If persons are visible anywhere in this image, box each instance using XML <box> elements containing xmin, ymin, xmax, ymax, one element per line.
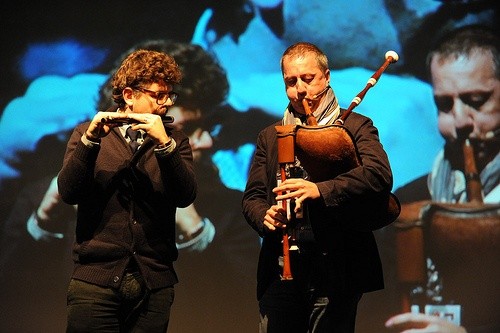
<box><xmin>56</xmin><ymin>50</ymin><xmax>196</xmax><ymax>332</ymax></box>
<box><xmin>243</xmin><ymin>42</ymin><xmax>393</xmax><ymax>332</ymax></box>
<box><xmin>0</xmin><ymin>38</ymin><xmax>283</xmax><ymax>333</ymax></box>
<box><xmin>352</xmin><ymin>0</ymin><xmax>500</xmax><ymax>333</ymax></box>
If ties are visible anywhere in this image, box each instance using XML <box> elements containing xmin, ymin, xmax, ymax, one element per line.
<box><xmin>127</xmin><ymin>126</ymin><xmax>140</xmax><ymax>155</ymax></box>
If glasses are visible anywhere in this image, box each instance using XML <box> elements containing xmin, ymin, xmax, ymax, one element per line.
<box><xmin>136</xmin><ymin>85</ymin><xmax>178</xmax><ymax>104</ymax></box>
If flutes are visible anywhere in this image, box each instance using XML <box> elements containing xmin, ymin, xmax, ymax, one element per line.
<box><xmin>396</xmin><ymin>138</ymin><xmax>500</xmax><ymax>333</ymax></box>
<box><xmin>275</xmin><ymin>50</ymin><xmax>399</xmax><ymax>282</ymax></box>
<box><xmin>101</xmin><ymin>116</ymin><xmax>175</xmax><ymax>124</ymax></box>
